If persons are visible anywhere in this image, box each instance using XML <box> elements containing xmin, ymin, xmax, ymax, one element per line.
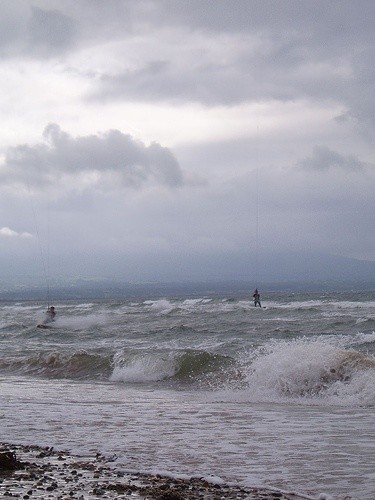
<box><xmin>253</xmin><ymin>289</ymin><xmax>262</xmax><ymax>307</ymax></box>
<box><xmin>40</xmin><ymin>307</ymin><xmax>56</xmax><ymax>326</ymax></box>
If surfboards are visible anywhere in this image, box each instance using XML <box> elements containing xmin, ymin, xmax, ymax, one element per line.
<box><xmin>36</xmin><ymin>324</ymin><xmax>45</xmax><ymax>328</ymax></box>
